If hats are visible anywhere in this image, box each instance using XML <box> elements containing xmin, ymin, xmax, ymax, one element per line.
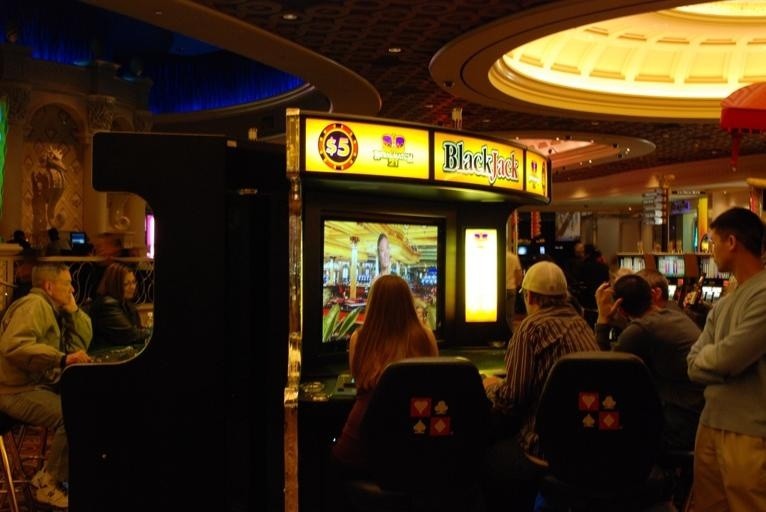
<box><xmin>519</xmin><ymin>261</ymin><xmax>568</xmax><ymax>295</ymax></box>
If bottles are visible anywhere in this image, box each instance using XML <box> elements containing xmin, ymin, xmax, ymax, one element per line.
<box><xmin>686</xmin><ymin>272</ymin><xmax>707</xmax><ymax>310</ymax></box>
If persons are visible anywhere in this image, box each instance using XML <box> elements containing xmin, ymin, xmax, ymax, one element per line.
<box><xmin>327</xmin><ymin>272</ymin><xmax>441</xmax><ymax>456</ymax></box>
<box><xmin>87</xmin><ymin>262</ymin><xmax>151</xmax><ymax>351</ymax></box>
<box><xmin>43</xmin><ymin>226</ymin><xmax>66</xmax><ymax>255</ymax></box>
<box><xmin>5</xmin><ymin>229</ymin><xmax>31</xmax><ymax>249</ymax></box>
<box><xmin>683</xmin><ymin>206</ymin><xmax>765</xmax><ymax>512</ymax></box>
<box><xmin>480</xmin><ymin>261</ymin><xmax>601</xmax><ymax>461</ymax></box>
<box><xmin>593</xmin><ymin>268</ymin><xmax>702</xmax><ymax>417</ymax></box>
<box><xmin>93</xmin><ymin>230</ymin><xmax>138</xmax><ymax>271</ymax></box>
<box><xmin>0</xmin><ymin>261</ymin><xmax>95</xmax><ymax>508</ymax></box>
<box><xmin>375</xmin><ymin>230</ymin><xmax>393</xmax><ymax>275</ymax></box>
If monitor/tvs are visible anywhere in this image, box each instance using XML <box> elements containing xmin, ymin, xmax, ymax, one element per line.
<box><xmin>323</xmin><ymin>214</ymin><xmax>445</xmax><ymax>349</ymax></box>
<box><xmin>70</xmin><ymin>233</ymin><xmax>85</xmax><ymax>248</ymax></box>
<box><xmin>462</xmin><ymin>225</ymin><xmax>499</xmax><ymax>323</ymax></box>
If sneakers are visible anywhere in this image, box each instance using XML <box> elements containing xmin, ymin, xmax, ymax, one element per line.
<box><xmin>31</xmin><ymin>469</ymin><xmax>69</xmax><ymax>508</ymax></box>
